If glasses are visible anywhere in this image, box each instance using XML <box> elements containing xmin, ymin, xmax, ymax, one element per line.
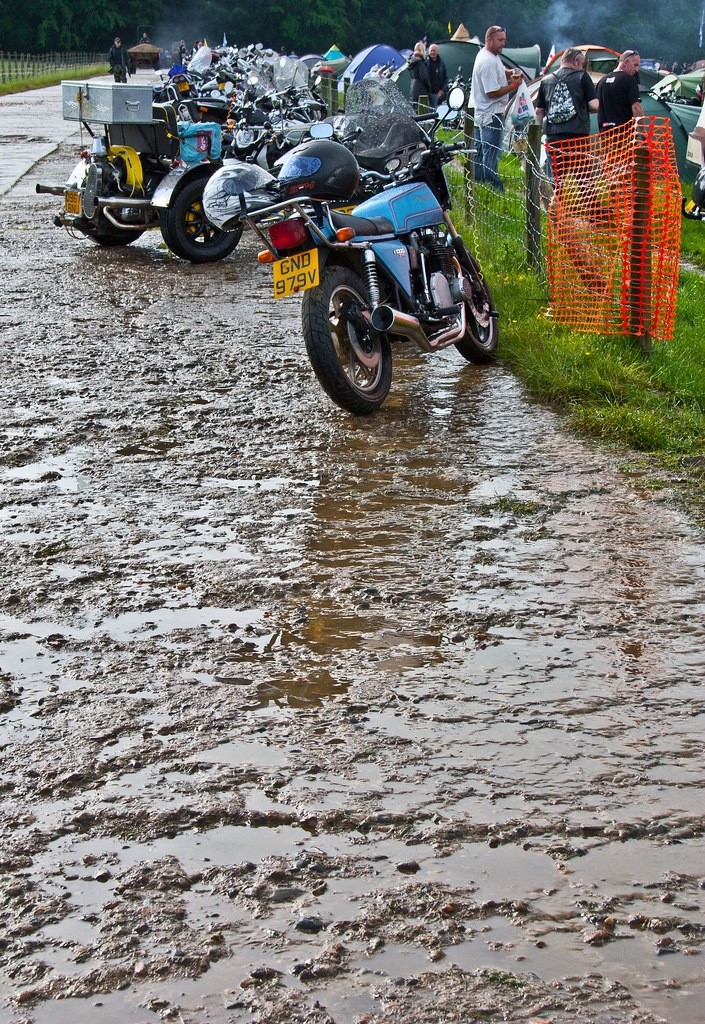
<box><xmin>622</xmin><ymin>49</ymin><xmax>639</xmax><ymax>62</ymax></box>
<box><xmin>561</xmin><ymin>49</ymin><xmax>582</xmax><ymax>64</ymax></box>
<box><xmin>486</xmin><ymin>27</ymin><xmax>506</xmax><ymax>38</ymax></box>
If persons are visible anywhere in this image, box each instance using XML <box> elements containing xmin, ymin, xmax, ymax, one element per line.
<box><xmin>178</xmin><ymin>40</ymin><xmax>186</xmax><ymax>66</ymax></box>
<box><xmin>108</xmin><ymin>37</ymin><xmax>132</xmax><ymax>83</ymax></box>
<box><xmin>289</xmin><ymin>54</ymin><xmax>298</xmax><ymax>59</ymax></box>
<box><xmin>594</xmin><ymin>50</ymin><xmax>646</xmax><ymax>209</ymax></box>
<box><xmin>425</xmin><ymin>44</ymin><xmax>449</xmax><ymax>106</ymax></box>
<box><xmin>535</xmin><ymin>45</ymin><xmax>616</xmax><ymax>226</ymax></box>
<box><xmin>139</xmin><ymin>32</ymin><xmax>150</xmax><ymax>45</ymax></box>
<box><xmin>348</xmin><ymin>54</ymin><xmax>353</xmax><ymax>61</ymax></box>
<box><xmin>468</xmin><ymin>24</ymin><xmax>524</xmax><ymax>193</ymax></box>
<box><xmin>409</xmin><ymin>42</ymin><xmax>436</xmax><ymax>112</ymax></box>
<box><xmin>192</xmin><ymin>39</ymin><xmax>199</xmax><ymax>54</ymax></box>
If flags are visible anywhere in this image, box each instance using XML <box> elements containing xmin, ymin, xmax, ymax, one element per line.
<box><xmin>223</xmin><ymin>34</ymin><xmax>228</xmax><ymax>47</ymax></box>
<box><xmin>203</xmin><ymin>39</ymin><xmax>207</xmax><ymax>46</ymax></box>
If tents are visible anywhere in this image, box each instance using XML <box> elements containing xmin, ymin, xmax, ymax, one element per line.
<box><xmin>649</xmin><ymin>69</ymin><xmax>705</xmax><ymax>108</ymax></box>
<box><xmin>503</xmin><ymin>67</ymin><xmax>701</xmax><ymax>192</ymax></box>
<box><xmin>451</xmin><ymin>23</ymin><xmax>482</xmax><ymax>46</ymax></box>
<box><xmin>311</xmin><ymin>56</ymin><xmax>352</xmax><ymax>82</ymax></box>
<box><xmin>337</xmin><ymin>43</ymin><xmax>408</xmax><ymax>110</ymax></box>
<box><xmin>299</xmin><ymin>52</ymin><xmax>327</xmax><ymax>67</ymax></box>
<box><xmin>390</xmin><ymin>38</ymin><xmax>542</xmax><ymax>122</ymax></box>
<box><xmin>543</xmin><ymin>42</ymin><xmax>625</xmax><ymax>76</ymax></box>
<box><xmin>399</xmin><ymin>47</ymin><xmax>414</xmax><ymax>57</ymax></box>
<box><xmin>323</xmin><ymin>43</ymin><xmax>348</xmax><ymax>61</ymax></box>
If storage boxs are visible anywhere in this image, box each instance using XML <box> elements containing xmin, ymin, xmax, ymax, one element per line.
<box><xmin>60</xmin><ymin>79</ymin><xmax>155</xmax><ymax>124</ymax></box>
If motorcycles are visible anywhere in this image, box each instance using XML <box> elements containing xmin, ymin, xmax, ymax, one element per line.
<box><xmin>241</xmin><ymin>82</ymin><xmax>506</xmax><ymax>414</ymax></box>
<box><xmin>35</xmin><ymin>33</ymin><xmax>462</xmax><ymax>263</ymax></box>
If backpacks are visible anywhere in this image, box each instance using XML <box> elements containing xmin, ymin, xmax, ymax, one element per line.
<box><xmin>546</xmin><ymin>67</ymin><xmax>583</xmax><ymax>124</ymax></box>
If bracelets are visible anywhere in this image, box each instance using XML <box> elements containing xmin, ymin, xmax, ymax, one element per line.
<box><xmin>509</xmin><ymin>82</ymin><xmax>515</xmax><ymax>90</ymax></box>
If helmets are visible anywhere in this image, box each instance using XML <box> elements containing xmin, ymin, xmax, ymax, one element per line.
<box><xmin>202</xmin><ymin>160</ymin><xmax>279</xmax><ymax>234</ymax></box>
<box><xmin>276</xmin><ymin>138</ymin><xmax>361</xmax><ymax>206</ymax></box>
